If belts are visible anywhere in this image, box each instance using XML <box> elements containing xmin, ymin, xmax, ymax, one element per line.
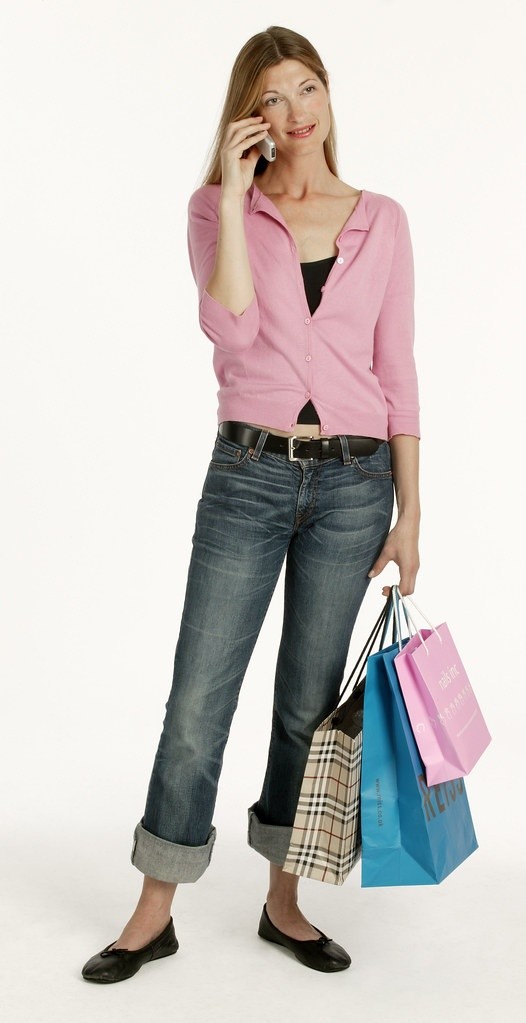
<box><xmin>218</xmin><ymin>422</ymin><xmax>381</xmax><ymax>460</ymax></box>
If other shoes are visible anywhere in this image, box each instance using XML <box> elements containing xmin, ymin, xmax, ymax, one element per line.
<box><xmin>81</xmin><ymin>914</ymin><xmax>180</xmax><ymax>983</ymax></box>
<box><xmin>257</xmin><ymin>903</ymin><xmax>352</xmax><ymax>973</ymax></box>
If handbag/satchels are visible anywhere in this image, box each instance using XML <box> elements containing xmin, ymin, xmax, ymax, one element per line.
<box><xmin>392</xmin><ymin>584</ymin><xmax>492</xmax><ymax>787</ymax></box>
<box><xmin>360</xmin><ymin>589</ymin><xmax>481</xmax><ymax>889</ymax></box>
<box><xmin>282</xmin><ymin>589</ymin><xmax>399</xmax><ymax>888</ymax></box>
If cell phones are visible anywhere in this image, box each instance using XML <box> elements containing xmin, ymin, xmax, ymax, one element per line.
<box><xmin>250</xmin><ymin>130</ymin><xmax>276</xmax><ymax>161</ymax></box>
